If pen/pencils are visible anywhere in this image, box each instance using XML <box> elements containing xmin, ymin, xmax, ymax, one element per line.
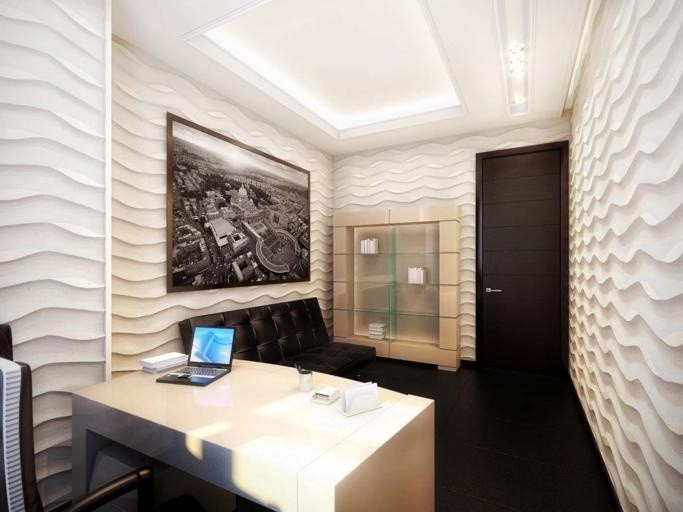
<box><xmin>294</xmin><ymin>363</ymin><xmax>302</xmax><ymax>373</ymax></box>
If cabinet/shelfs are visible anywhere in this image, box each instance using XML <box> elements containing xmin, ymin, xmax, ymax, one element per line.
<box><xmin>333</xmin><ymin>205</ymin><xmax>461</xmax><ymax>372</ymax></box>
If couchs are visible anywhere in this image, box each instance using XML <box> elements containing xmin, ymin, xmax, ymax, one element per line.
<box><xmin>178</xmin><ymin>296</ymin><xmax>376</xmax><ymax>377</ymax></box>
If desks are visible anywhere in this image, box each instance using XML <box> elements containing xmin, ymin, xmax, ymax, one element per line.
<box><xmin>71</xmin><ymin>358</ymin><xmax>437</xmax><ymax>512</ymax></box>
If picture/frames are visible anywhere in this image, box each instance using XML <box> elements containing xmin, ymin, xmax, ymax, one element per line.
<box><xmin>167</xmin><ymin>112</ymin><xmax>310</xmax><ymax>293</ymax></box>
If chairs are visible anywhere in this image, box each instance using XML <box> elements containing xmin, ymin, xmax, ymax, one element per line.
<box><xmin>1</xmin><ymin>323</ymin><xmax>205</xmax><ymax>512</ymax></box>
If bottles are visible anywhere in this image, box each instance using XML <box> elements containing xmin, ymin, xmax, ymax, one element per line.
<box><xmin>296</xmin><ymin>363</ymin><xmax>313</xmax><ymax>391</ymax></box>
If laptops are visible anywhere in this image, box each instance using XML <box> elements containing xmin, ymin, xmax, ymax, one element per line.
<box><xmin>155</xmin><ymin>326</ymin><xmax>237</xmax><ymax>386</ymax></box>
